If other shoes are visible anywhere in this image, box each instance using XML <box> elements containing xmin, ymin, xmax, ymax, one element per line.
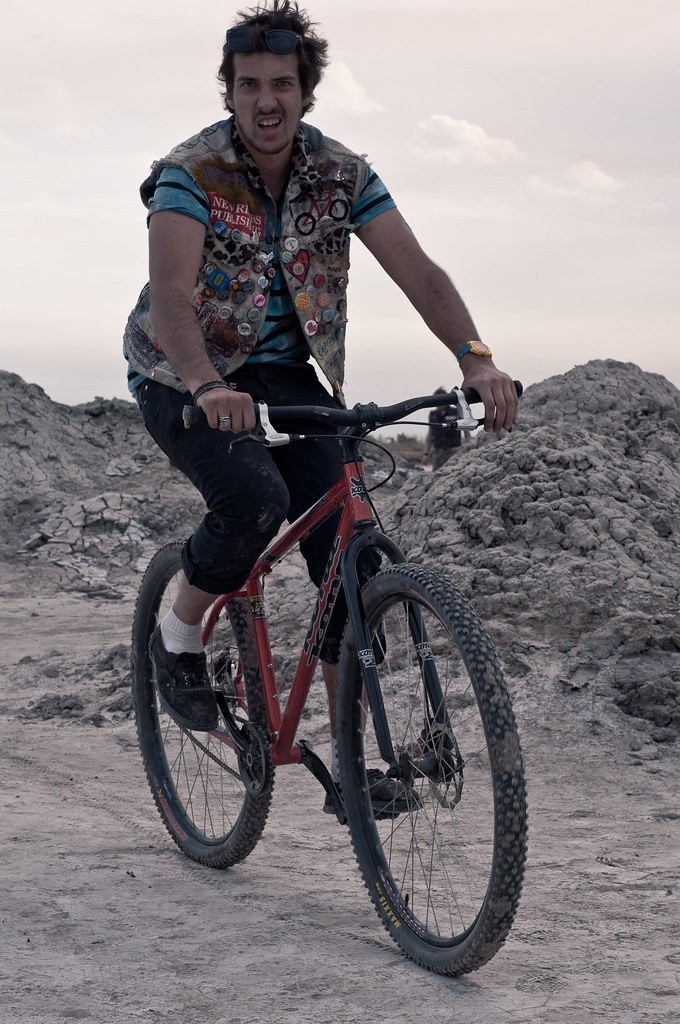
<box><xmin>148</xmin><ymin>623</ymin><xmax>219</xmax><ymax>731</ymax></box>
<box><xmin>322</xmin><ymin>769</ymin><xmax>424</xmax><ymax>813</ymax></box>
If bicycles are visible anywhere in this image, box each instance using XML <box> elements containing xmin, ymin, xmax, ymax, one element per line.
<box><xmin>130</xmin><ymin>380</ymin><xmax>527</xmax><ymax>977</ymax></box>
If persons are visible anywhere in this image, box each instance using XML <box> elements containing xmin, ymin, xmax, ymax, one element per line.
<box><xmin>421</xmin><ymin>387</ymin><xmax>463</xmax><ymax>471</ymax></box>
<box><xmin>122</xmin><ymin>0</ymin><xmax>518</xmax><ymax>813</ymax></box>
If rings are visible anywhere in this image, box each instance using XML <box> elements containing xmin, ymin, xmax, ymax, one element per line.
<box><xmin>219</xmin><ymin>417</ymin><xmax>232</xmax><ymax>424</ymax></box>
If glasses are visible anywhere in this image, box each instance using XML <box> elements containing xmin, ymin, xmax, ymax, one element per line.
<box><xmin>226</xmin><ymin>28</ymin><xmax>302</xmax><ymax>57</ymax></box>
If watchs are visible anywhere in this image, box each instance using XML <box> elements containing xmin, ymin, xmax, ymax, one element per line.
<box><xmin>456</xmin><ymin>341</ymin><xmax>492</xmax><ymax>359</ymax></box>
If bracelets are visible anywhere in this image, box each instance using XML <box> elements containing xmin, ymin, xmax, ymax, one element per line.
<box><xmin>194</xmin><ymin>380</ymin><xmax>233</xmax><ymax>403</ymax></box>
<box><xmin>424</xmin><ymin>453</ymin><xmax>428</xmax><ymax>456</ymax></box>
